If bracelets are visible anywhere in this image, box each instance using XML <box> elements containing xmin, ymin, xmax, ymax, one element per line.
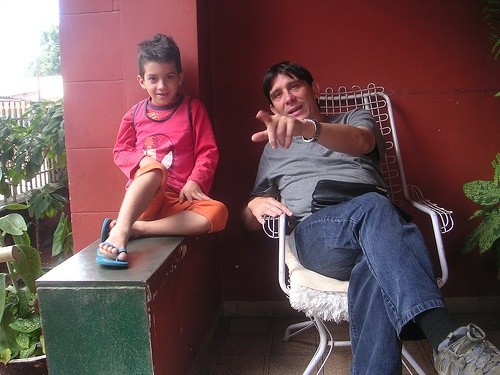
<box><xmin>301</xmin><ymin>119</ymin><xmax>321</xmax><ymax>143</ymax></box>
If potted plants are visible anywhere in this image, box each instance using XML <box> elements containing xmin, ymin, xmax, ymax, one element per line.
<box><xmin>0</xmin><ymin>204</ymin><xmax>49</xmax><ymax>375</ymax></box>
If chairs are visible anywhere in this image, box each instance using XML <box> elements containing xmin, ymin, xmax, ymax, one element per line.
<box><xmin>262</xmin><ymin>82</ymin><xmax>454</xmax><ymax>375</ymax></box>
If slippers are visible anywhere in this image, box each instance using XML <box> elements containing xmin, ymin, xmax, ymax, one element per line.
<box><xmin>101</xmin><ymin>217</ymin><xmax>115</xmax><ymax>242</ymax></box>
<box><xmin>96</xmin><ymin>241</ymin><xmax>129</xmax><ymax>267</ymax></box>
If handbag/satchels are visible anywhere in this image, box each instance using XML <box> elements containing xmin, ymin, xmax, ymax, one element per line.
<box><xmin>311</xmin><ymin>180</ymin><xmax>389</xmax><ymax>213</ymax></box>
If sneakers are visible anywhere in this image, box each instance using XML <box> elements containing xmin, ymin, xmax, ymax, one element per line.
<box><xmin>430</xmin><ymin>323</ymin><xmax>500</xmax><ymax>374</ymax></box>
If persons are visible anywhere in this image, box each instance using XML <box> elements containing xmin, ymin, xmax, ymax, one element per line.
<box><xmin>95</xmin><ymin>33</ymin><xmax>228</xmax><ymax>267</ymax></box>
<box><xmin>241</xmin><ymin>59</ymin><xmax>500</xmax><ymax>375</ymax></box>
